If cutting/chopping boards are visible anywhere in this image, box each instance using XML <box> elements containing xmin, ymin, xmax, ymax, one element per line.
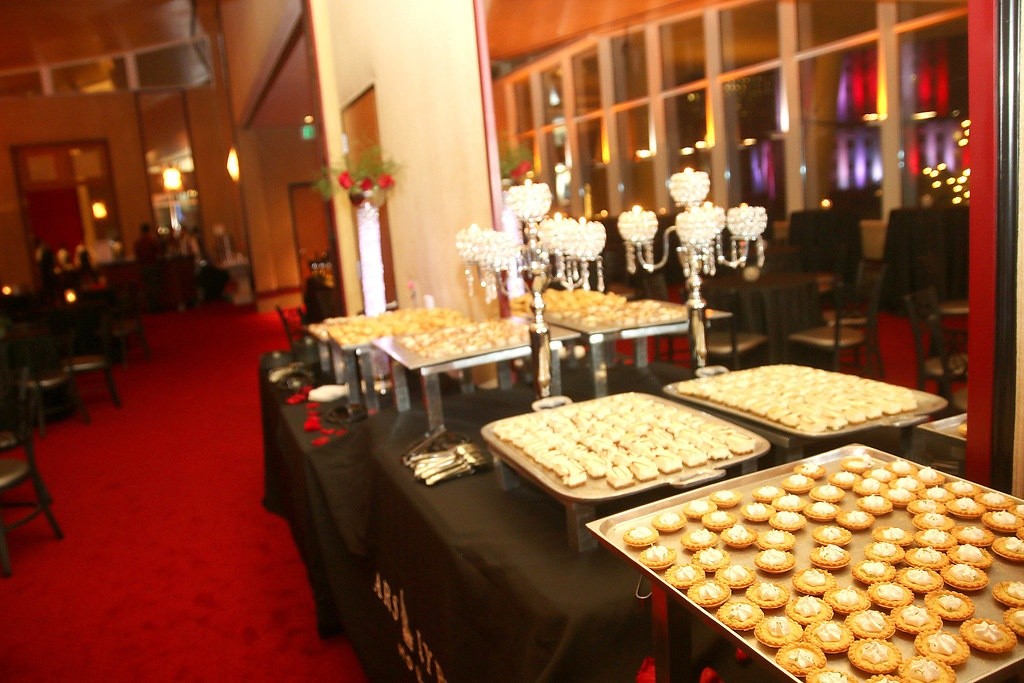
<box><xmin>481</xmin><ymin>391</ymin><xmax>771</xmax><ymax>501</ymax></box>
<box><xmin>664</xmin><ymin>364</ymin><xmax>948</xmax><ymax>437</ymax></box>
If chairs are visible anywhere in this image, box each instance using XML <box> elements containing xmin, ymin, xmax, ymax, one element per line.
<box><xmin>600</xmin><ymin>200</ymin><xmax>980</xmax><ymax>416</ymax></box>
<box><xmin>0</xmin><ymin>282</ymin><xmax>147</xmax><ymax>577</ymax></box>
<box><xmin>274</xmin><ymin>303</ymin><xmax>305</xmax><ymax>345</ymax></box>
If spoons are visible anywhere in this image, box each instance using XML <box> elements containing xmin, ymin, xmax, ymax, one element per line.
<box><xmin>407</xmin><ymin>444</ymin><xmax>487</xmax><ymax>485</ymax></box>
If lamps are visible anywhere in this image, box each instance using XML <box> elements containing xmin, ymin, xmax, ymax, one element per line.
<box><xmin>623</xmin><ymin>163</ymin><xmax>768</xmax><ymax>375</ymax></box>
<box><xmin>456</xmin><ymin>165</ymin><xmax>607</xmax><ymax>410</ymax></box>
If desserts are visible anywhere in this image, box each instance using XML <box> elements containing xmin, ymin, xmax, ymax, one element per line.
<box><xmin>676</xmin><ymin>365</ymin><xmax>920</xmax><ymax>432</ymax></box>
<box><xmin>491</xmin><ymin>391</ymin><xmax>754</xmax><ymax>491</ymax></box>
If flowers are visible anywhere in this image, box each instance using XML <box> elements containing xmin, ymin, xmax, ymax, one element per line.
<box><xmin>316</xmin><ymin>147</ymin><xmax>404</xmax><ymax>209</ymax></box>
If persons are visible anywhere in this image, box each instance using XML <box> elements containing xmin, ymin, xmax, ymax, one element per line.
<box><xmin>28</xmin><ymin>222</ymin><xmax>157</xmax><ymax>317</ymax></box>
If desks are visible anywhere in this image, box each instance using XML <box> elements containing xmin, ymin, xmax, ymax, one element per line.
<box><xmin>260</xmin><ymin>327</ymin><xmax>828</xmax><ymax>683</ymax></box>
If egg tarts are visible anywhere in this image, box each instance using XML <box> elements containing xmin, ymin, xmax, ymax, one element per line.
<box><xmin>624</xmin><ymin>454</ymin><xmax>1024</xmax><ymax>683</ymax></box>
